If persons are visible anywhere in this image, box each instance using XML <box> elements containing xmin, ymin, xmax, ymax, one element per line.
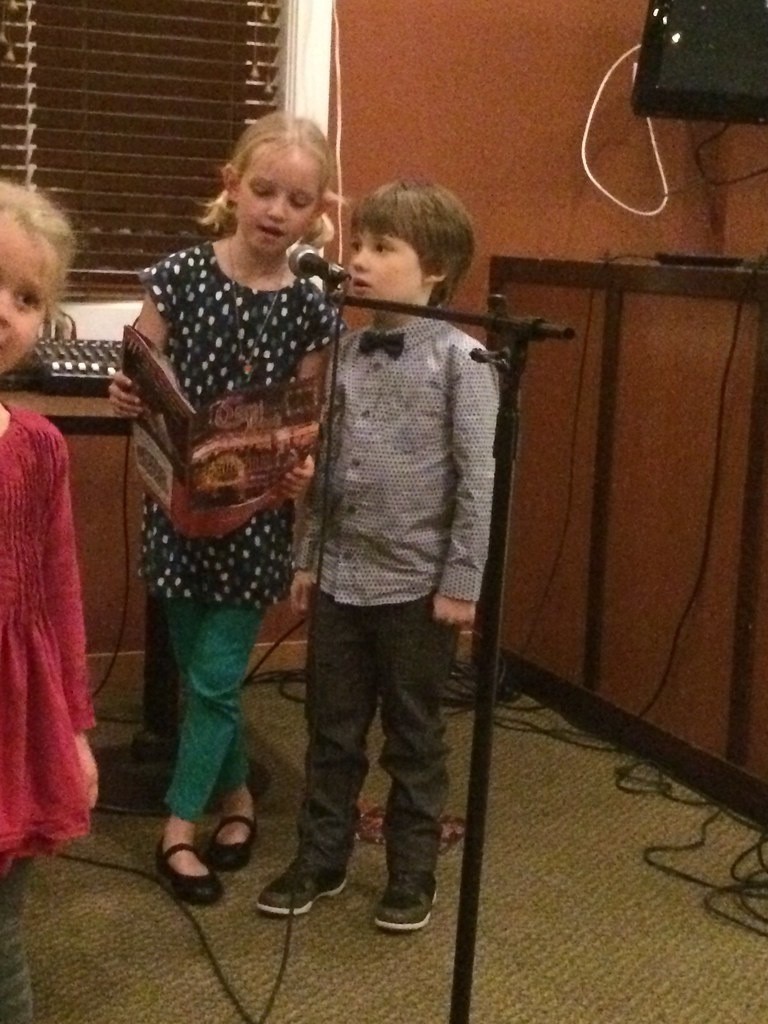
<box><xmin>0</xmin><ymin>177</ymin><xmax>98</xmax><ymax>1024</ymax></box>
<box><xmin>106</xmin><ymin>111</ymin><xmax>347</xmax><ymax>905</ymax></box>
<box><xmin>252</xmin><ymin>179</ymin><xmax>498</xmax><ymax>936</ymax></box>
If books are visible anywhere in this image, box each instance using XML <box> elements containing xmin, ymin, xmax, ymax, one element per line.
<box><xmin>123</xmin><ymin>323</ymin><xmax>329</xmax><ymax>539</ymax></box>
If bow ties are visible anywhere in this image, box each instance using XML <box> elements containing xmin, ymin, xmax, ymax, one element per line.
<box><xmin>354</xmin><ymin>329</ymin><xmax>403</xmax><ymax>358</ymax></box>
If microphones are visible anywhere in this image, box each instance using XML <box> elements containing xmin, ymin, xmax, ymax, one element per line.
<box><xmin>288</xmin><ymin>244</ymin><xmax>351</xmax><ymax>283</ymax></box>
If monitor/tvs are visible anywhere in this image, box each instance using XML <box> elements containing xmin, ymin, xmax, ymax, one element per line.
<box><xmin>630</xmin><ymin>2</ymin><xmax>767</xmax><ymax>125</ymax></box>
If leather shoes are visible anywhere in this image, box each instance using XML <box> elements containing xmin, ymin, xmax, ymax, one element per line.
<box><xmin>157</xmin><ymin>836</ymin><xmax>224</xmax><ymax>904</ymax></box>
<box><xmin>210</xmin><ymin>810</ymin><xmax>255</xmax><ymax>870</ymax></box>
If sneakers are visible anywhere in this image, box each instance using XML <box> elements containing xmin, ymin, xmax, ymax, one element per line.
<box><xmin>259</xmin><ymin>859</ymin><xmax>349</xmax><ymax>917</ymax></box>
<box><xmin>374</xmin><ymin>876</ymin><xmax>438</xmax><ymax>929</ymax></box>
